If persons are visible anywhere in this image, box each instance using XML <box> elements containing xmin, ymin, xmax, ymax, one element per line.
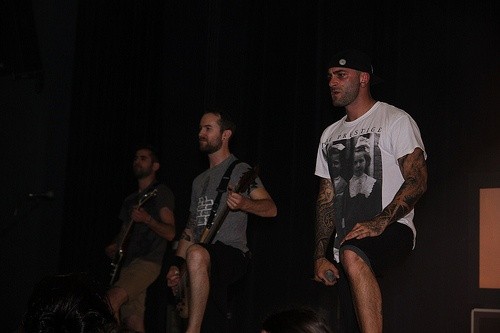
<box><xmin>24</xmin><ymin>272</ymin><xmax>120</xmax><ymax>333</ymax></box>
<box><xmin>166</xmin><ymin>106</ymin><xmax>278</xmax><ymax>333</ymax></box>
<box><xmin>101</xmin><ymin>146</ymin><xmax>176</xmax><ymax>333</ymax></box>
<box><xmin>258</xmin><ymin>305</ymin><xmax>331</xmax><ymax>333</ymax></box>
<box><xmin>311</xmin><ymin>52</ymin><xmax>428</xmax><ymax>333</ymax></box>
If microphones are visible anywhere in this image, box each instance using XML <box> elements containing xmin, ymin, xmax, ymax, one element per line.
<box><xmin>325</xmin><ymin>271</ymin><xmax>335</xmax><ymax>282</ymax></box>
<box><xmin>29</xmin><ymin>191</ymin><xmax>54</xmax><ymax>199</ymax></box>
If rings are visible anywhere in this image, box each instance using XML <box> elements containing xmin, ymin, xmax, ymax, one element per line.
<box><xmin>134</xmin><ymin>215</ymin><xmax>137</xmax><ymax>218</ymax></box>
<box><xmin>365</xmin><ymin>233</ymin><xmax>370</xmax><ymax>237</ymax></box>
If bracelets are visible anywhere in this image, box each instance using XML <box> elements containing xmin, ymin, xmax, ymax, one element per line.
<box><xmin>313</xmin><ymin>255</ymin><xmax>324</xmax><ymax>260</ymax></box>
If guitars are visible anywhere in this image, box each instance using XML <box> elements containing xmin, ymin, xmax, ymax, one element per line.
<box><xmin>172</xmin><ymin>164</ymin><xmax>262</xmax><ymax>318</ymax></box>
<box><xmin>104</xmin><ymin>188</ymin><xmax>158</xmax><ymax>284</ymax></box>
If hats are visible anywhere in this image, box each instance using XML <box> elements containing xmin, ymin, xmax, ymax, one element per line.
<box><xmin>329</xmin><ymin>53</ymin><xmax>369</xmax><ymax>74</ymax></box>
